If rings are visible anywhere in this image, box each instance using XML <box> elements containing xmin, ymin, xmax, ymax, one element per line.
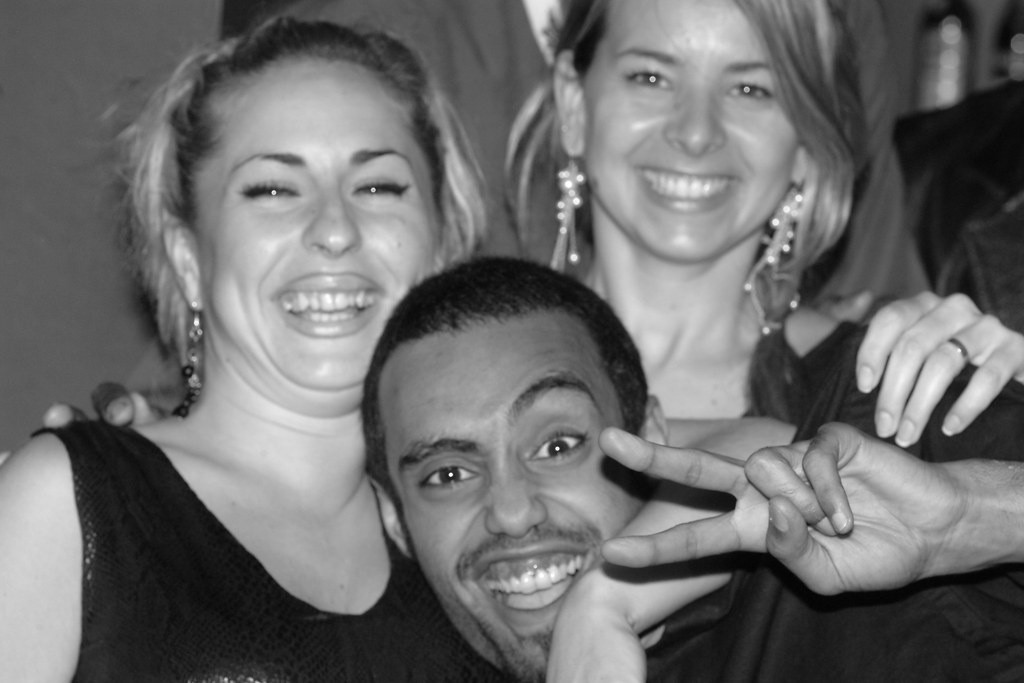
<box><xmin>947</xmin><ymin>338</ymin><xmax>969</xmax><ymax>363</ymax></box>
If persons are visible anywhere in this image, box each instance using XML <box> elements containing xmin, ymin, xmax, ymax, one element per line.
<box><xmin>41</xmin><ymin>0</ymin><xmax>1024</xmax><ymax>683</ymax></box>
<box><xmin>0</xmin><ymin>15</ymin><xmax>808</xmax><ymax>683</ymax></box>
